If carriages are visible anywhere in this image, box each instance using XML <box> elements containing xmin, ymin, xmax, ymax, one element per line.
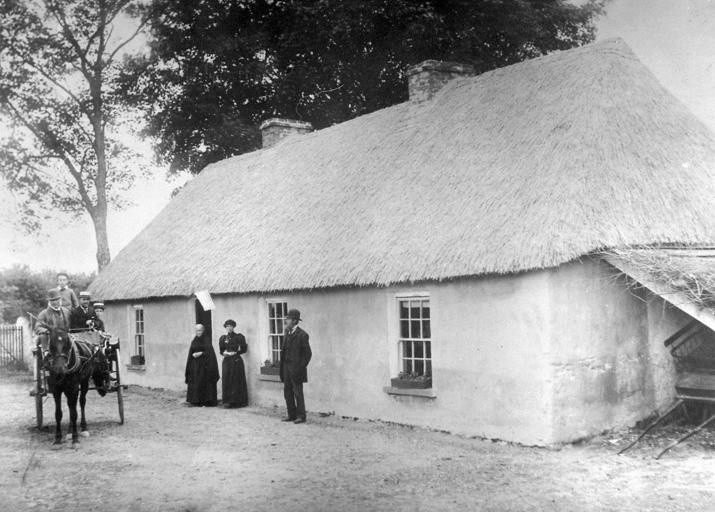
<box><xmin>31</xmin><ymin>328</ymin><xmax>125</xmax><ymax>447</ymax></box>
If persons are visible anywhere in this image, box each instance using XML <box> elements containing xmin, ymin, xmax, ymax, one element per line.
<box><xmin>72</xmin><ymin>291</ymin><xmax>104</xmax><ymax>332</ymax></box>
<box><xmin>184</xmin><ymin>323</ymin><xmax>220</xmax><ymax>407</ymax></box>
<box><xmin>34</xmin><ymin>287</ymin><xmax>72</xmax><ymax>336</ymax></box>
<box><xmin>56</xmin><ymin>272</ymin><xmax>79</xmax><ymax>310</ymax></box>
<box><xmin>278</xmin><ymin>308</ymin><xmax>314</xmax><ymax>425</ymax></box>
<box><xmin>92</xmin><ymin>302</ymin><xmax>106</xmax><ymax>320</ymax></box>
<box><xmin>218</xmin><ymin>318</ymin><xmax>248</xmax><ymax>409</ymax></box>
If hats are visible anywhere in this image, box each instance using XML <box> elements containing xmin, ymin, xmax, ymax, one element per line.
<box><xmin>44</xmin><ymin>288</ymin><xmax>61</xmax><ymax>301</ymax></box>
<box><xmin>285</xmin><ymin>309</ymin><xmax>302</xmax><ymax>321</ymax></box>
<box><xmin>223</xmin><ymin>319</ymin><xmax>236</xmax><ymax>328</ymax></box>
<box><xmin>92</xmin><ymin>302</ymin><xmax>106</xmax><ymax>310</ymax></box>
<box><xmin>77</xmin><ymin>291</ymin><xmax>92</xmax><ymax>300</ymax></box>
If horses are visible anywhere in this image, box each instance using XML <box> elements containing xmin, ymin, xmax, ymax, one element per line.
<box><xmin>44</xmin><ymin>324</ymin><xmax>94</xmax><ymax>450</ymax></box>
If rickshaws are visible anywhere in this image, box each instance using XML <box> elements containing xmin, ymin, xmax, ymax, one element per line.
<box><xmin>617</xmin><ymin>318</ymin><xmax>715</xmax><ymax>465</ymax></box>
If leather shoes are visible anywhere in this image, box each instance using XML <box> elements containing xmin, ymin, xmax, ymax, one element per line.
<box><xmin>281</xmin><ymin>416</ymin><xmax>306</xmax><ymax>423</ymax></box>
